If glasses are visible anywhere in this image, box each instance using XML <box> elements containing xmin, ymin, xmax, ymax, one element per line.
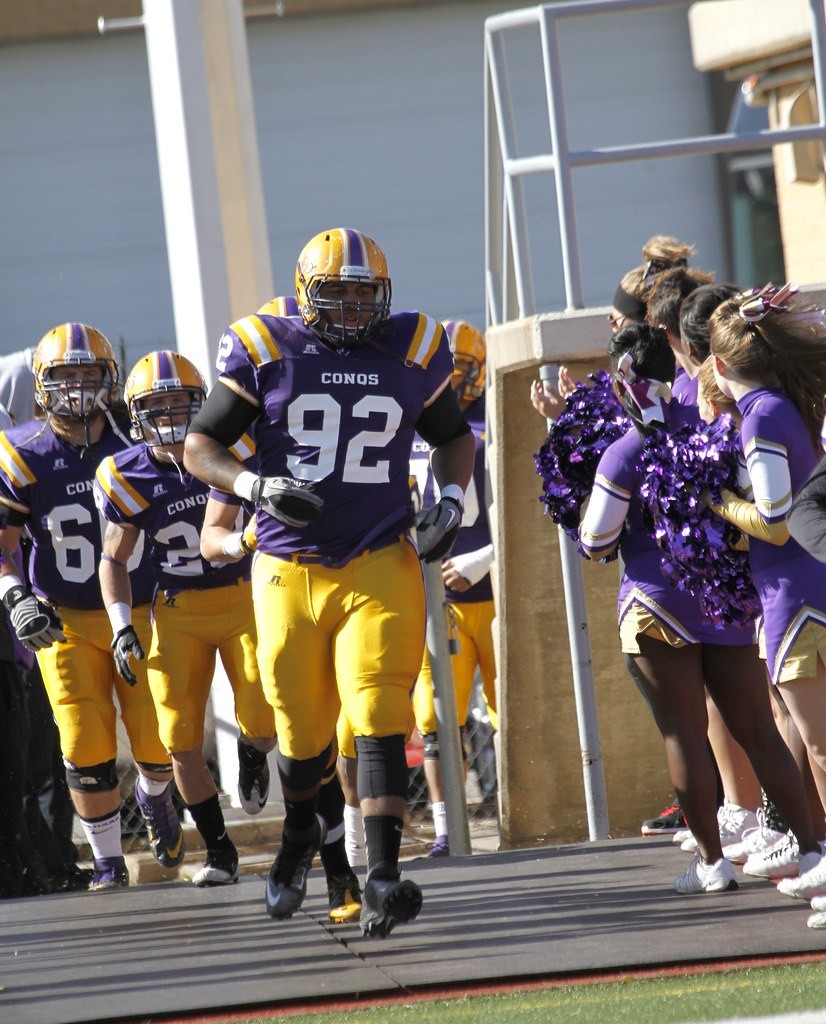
<box><xmin>606</xmin><ymin>314</ymin><xmax>624</xmax><ymax>333</ymax></box>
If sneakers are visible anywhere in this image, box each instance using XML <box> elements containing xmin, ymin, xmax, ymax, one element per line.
<box><xmin>88</xmin><ymin>856</ymin><xmax>130</xmax><ymax>891</ymax></box>
<box><xmin>134</xmin><ymin>775</ymin><xmax>187</xmax><ymax>868</ymax></box>
<box><xmin>424</xmin><ymin>834</ymin><xmax>449</xmax><ymax>857</ymax></box>
<box><xmin>672</xmin><ymin>800</ymin><xmax>826</xmax><ymax>930</ymax></box>
<box><xmin>191</xmin><ymin>849</ymin><xmax>240</xmax><ymax>888</ymax></box>
<box><xmin>673</xmin><ymin>851</ymin><xmax>740</xmax><ymax>894</ymax></box>
<box><xmin>640</xmin><ymin>802</ymin><xmax>691</xmax><ymax>836</ymax></box>
<box><xmin>357</xmin><ymin>876</ymin><xmax>424</xmax><ymax>940</ymax></box>
<box><xmin>264</xmin><ymin>812</ymin><xmax>328</xmax><ymax>920</ymax></box>
<box><xmin>235</xmin><ymin>732</ymin><xmax>271</xmax><ymax>815</ymax></box>
<box><xmin>323</xmin><ymin>873</ymin><xmax>362</xmax><ymax>925</ymax></box>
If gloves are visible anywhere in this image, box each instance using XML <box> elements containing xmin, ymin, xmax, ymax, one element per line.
<box><xmin>415</xmin><ymin>496</ymin><xmax>465</xmax><ymax>565</ymax></box>
<box><xmin>239</xmin><ymin>513</ymin><xmax>257</xmax><ymax>555</ymax></box>
<box><xmin>0</xmin><ymin>585</ymin><xmax>68</xmax><ymax>655</ymax></box>
<box><xmin>249</xmin><ymin>475</ymin><xmax>324</xmax><ymax>529</ymax></box>
<box><xmin>109</xmin><ymin>624</ymin><xmax>145</xmax><ymax>688</ymax></box>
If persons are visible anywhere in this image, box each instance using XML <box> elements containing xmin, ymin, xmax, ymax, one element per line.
<box><xmin>197</xmin><ymin>290</ymin><xmax>500</xmax><ymax>922</ymax></box>
<box><xmin>94</xmin><ymin>348</ymin><xmax>275</xmax><ymax>886</ymax></box>
<box><xmin>526</xmin><ymin>236</ymin><xmax>826</xmax><ymax>929</ymax></box>
<box><xmin>184</xmin><ymin>227</ymin><xmax>479</xmax><ymax>934</ymax></box>
<box><xmin>0</xmin><ymin>321</ymin><xmax>193</xmax><ymax>900</ymax></box>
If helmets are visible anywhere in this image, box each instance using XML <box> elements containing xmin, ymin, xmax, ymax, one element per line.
<box><xmin>257</xmin><ymin>295</ymin><xmax>300</xmax><ymax>318</ymax></box>
<box><xmin>440</xmin><ymin>319</ymin><xmax>487</xmax><ymax>410</ymax></box>
<box><xmin>28</xmin><ymin>321</ymin><xmax>121</xmax><ymax>411</ymax></box>
<box><xmin>121</xmin><ymin>349</ymin><xmax>207</xmax><ymax>440</ymax></box>
<box><xmin>291</xmin><ymin>227</ymin><xmax>393</xmax><ymax>328</ymax></box>
